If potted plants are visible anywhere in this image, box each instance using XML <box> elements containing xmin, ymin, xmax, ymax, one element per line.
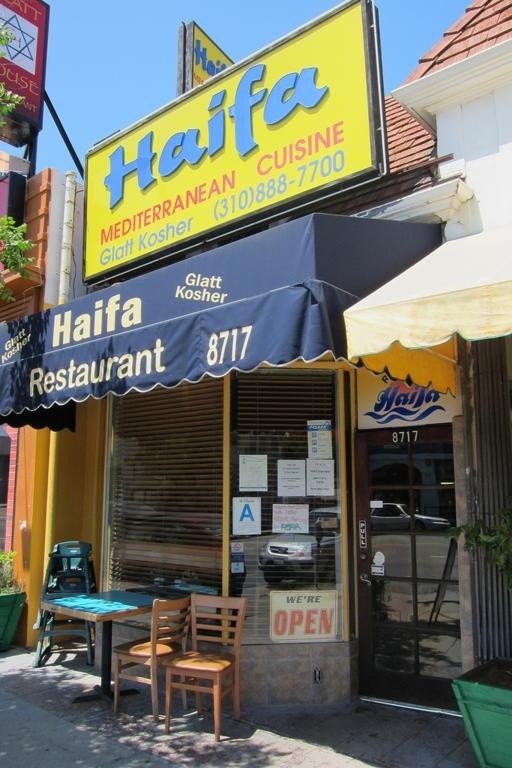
<box><xmin>0</xmin><ymin>214</ymin><xmax>44</xmax><ymax>301</ymax></box>
<box><xmin>445</xmin><ymin>515</ymin><xmax>512</xmax><ymax>768</ymax></box>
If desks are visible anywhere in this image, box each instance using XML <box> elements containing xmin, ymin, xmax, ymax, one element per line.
<box><xmin>41</xmin><ymin>586</ymin><xmax>190</xmax><ymax>708</ymax></box>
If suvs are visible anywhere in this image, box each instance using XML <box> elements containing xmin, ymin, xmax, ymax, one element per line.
<box><xmin>257</xmin><ymin>505</ymin><xmax>340</xmax><ymax>585</ymax></box>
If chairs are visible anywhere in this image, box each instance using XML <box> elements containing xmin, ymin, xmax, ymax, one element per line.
<box><xmin>161</xmin><ymin>591</ymin><xmax>249</xmax><ymax>741</ymax></box>
<box><xmin>111</xmin><ymin>596</ymin><xmax>191</xmax><ymax>721</ymax></box>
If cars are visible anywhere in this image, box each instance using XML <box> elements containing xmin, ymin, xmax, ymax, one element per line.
<box><xmin>109</xmin><ymin>499</ymin><xmax>155</xmax><ymax>525</ymax></box>
<box><xmin>109</xmin><ymin>510</ymin><xmax>247</xmax><ymax>598</ymax></box>
<box><xmin>369</xmin><ymin>502</ymin><xmax>450</xmax><ymax>534</ymax></box>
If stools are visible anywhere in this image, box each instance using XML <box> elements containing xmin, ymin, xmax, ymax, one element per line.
<box><xmin>32</xmin><ymin>541</ymin><xmax>97</xmax><ymax>668</ymax></box>
